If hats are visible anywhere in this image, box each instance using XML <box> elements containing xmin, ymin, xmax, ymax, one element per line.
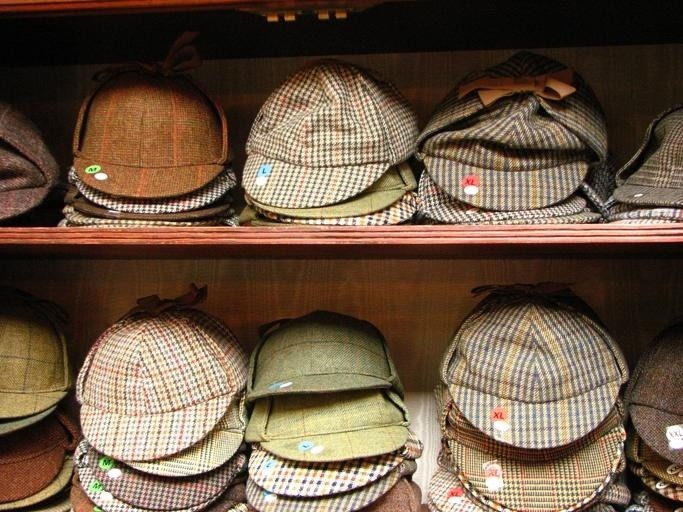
<box><xmin>0</xmin><ymin>282</ymin><xmax>79</xmax><ymax>421</ymax></box>
<box><xmin>612</xmin><ymin>99</ymin><xmax>683</xmax><ymax>212</ymax></box>
<box><xmin>438</xmin><ymin>280</ymin><xmax>624</xmax><ymax>450</ymax></box>
<box><xmin>622</xmin><ymin>318</ymin><xmax>683</xmax><ymax>468</ymax></box>
<box><xmin>244</xmin><ymin>307</ymin><xmax>406</xmax><ymax>411</ymax></box>
<box><xmin>0</xmin><ymin>92</ymin><xmax>64</xmax><ymax>223</ymax></box>
<box><xmin>239</xmin><ymin>160</ymin><xmax>417</xmax><ymax>225</ymax></box>
<box><xmin>58</xmin><ymin>167</ymin><xmax>239</xmax><ymax>228</ymax></box>
<box><xmin>1</xmin><ymin>403</ymin><xmax>76</xmax><ymax>512</ymax></box>
<box><xmin>70</xmin><ymin>392</ymin><xmax>249</xmax><ymax>512</ymax></box>
<box><xmin>74</xmin><ymin>283</ymin><xmax>251</xmax><ymax>464</ymax></box>
<box><xmin>606</xmin><ymin>194</ymin><xmax>683</xmax><ymax>225</ymax></box>
<box><xmin>417</xmin><ymin>166</ymin><xmax>613</xmax><ymax>233</ymax></box>
<box><xmin>241</xmin><ymin>58</ymin><xmax>421</xmax><ymax>211</ymax></box>
<box><xmin>626</xmin><ymin>430</ymin><xmax>683</xmax><ymax>511</ymax></box>
<box><xmin>412</xmin><ymin>51</ymin><xmax>606</xmax><ymax>210</ymax></box>
<box><xmin>245</xmin><ymin>389</ymin><xmax>422</xmax><ymax>512</ymax></box>
<box><xmin>427</xmin><ymin>381</ymin><xmax>632</xmax><ymax>511</ymax></box>
<box><xmin>72</xmin><ymin>49</ymin><xmax>235</xmax><ymax>200</ymax></box>
